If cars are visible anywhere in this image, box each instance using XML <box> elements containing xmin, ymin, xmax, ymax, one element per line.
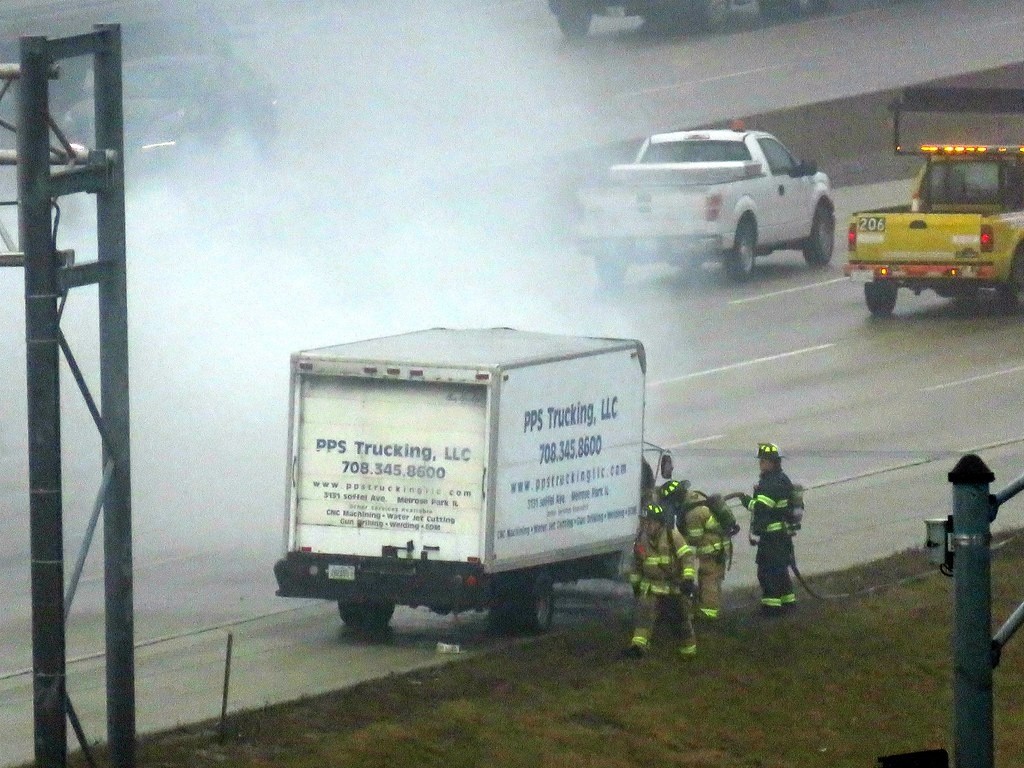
<box><xmin>57</xmin><ymin>56</ymin><xmax>253</xmax><ymax>186</ymax></box>
<box><xmin>548</xmin><ymin>0</ymin><xmax>829</xmax><ymax>39</ymax></box>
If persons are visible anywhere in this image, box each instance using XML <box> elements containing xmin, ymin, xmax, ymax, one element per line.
<box><xmin>657</xmin><ymin>479</ymin><xmax>732</xmax><ymax>621</ymax></box>
<box><xmin>739</xmin><ymin>443</ymin><xmax>796</xmax><ymax>614</ymax></box>
<box><xmin>620</xmin><ymin>502</ymin><xmax>698</xmax><ymax>661</ymax></box>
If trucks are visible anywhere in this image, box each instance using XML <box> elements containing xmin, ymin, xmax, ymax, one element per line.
<box><xmin>273</xmin><ymin>325</ymin><xmax>676</xmax><ymax>641</ymax></box>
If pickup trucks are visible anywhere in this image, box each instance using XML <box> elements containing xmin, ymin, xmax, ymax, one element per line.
<box><xmin>841</xmin><ymin>143</ymin><xmax>1024</xmax><ymax>316</ymax></box>
<box><xmin>592</xmin><ymin>119</ymin><xmax>838</xmax><ymax>297</ymax></box>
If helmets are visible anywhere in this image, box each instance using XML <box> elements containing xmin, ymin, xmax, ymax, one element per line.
<box><xmin>641</xmin><ymin>502</ymin><xmax>667</xmax><ymax>521</ymax></box>
<box><xmin>755</xmin><ymin>443</ymin><xmax>787</xmax><ymax>459</ymax></box>
<box><xmin>656</xmin><ymin>479</ymin><xmax>686</xmax><ymax>505</ymax></box>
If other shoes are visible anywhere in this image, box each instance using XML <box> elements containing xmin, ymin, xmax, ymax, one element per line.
<box><xmin>677</xmin><ymin>649</ymin><xmax>693</xmax><ymax>662</ymax></box>
<box><xmin>627</xmin><ymin>643</ymin><xmax>645</xmax><ymax>659</ymax></box>
<box><xmin>758</xmin><ymin>604</ymin><xmax>781</xmax><ymax>616</ymax></box>
<box><xmin>780</xmin><ymin>602</ymin><xmax>797</xmax><ymax>617</ymax></box>
